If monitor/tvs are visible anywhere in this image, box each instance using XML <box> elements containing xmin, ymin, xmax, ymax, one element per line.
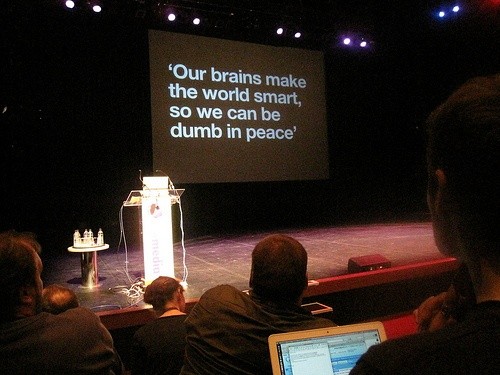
<box><xmin>143</xmin><ymin>177</ymin><xmax>168</xmax><ymax>190</ymax></box>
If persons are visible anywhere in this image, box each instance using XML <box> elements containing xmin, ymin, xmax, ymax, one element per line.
<box><xmin>348</xmin><ymin>74</ymin><xmax>500</xmax><ymax>375</ymax></box>
<box><xmin>0</xmin><ymin>233</ymin><xmax>339</xmax><ymax>375</ymax></box>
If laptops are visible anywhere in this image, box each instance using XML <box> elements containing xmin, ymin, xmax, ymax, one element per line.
<box><xmin>267</xmin><ymin>321</ymin><xmax>388</xmax><ymax>375</ymax></box>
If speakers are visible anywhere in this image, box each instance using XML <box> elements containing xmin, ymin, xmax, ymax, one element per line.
<box><xmin>347</xmin><ymin>254</ymin><xmax>391</xmax><ymax>274</ymax></box>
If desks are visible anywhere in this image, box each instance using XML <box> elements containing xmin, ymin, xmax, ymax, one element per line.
<box><xmin>67</xmin><ymin>244</ymin><xmax>109</xmax><ymax>287</ymax></box>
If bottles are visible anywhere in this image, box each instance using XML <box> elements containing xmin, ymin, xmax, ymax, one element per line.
<box><xmin>88</xmin><ymin>229</ymin><xmax>93</xmax><ymax>246</ymax></box>
<box><xmin>98</xmin><ymin>228</ymin><xmax>104</xmax><ymax>245</ymax></box>
<box><xmin>73</xmin><ymin>229</ymin><xmax>81</xmax><ymax>247</ymax></box>
<box><xmin>83</xmin><ymin>229</ymin><xmax>88</xmax><ymax>246</ymax></box>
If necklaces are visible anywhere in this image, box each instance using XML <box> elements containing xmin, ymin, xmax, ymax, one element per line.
<box><xmin>162</xmin><ymin>306</ymin><xmax>179</xmax><ymax>314</ymax></box>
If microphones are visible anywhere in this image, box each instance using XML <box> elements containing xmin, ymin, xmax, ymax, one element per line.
<box><xmin>156</xmin><ymin>170</ymin><xmax>174</xmax><ymax>189</ymax></box>
<box><xmin>139</xmin><ymin>170</ymin><xmax>150</xmax><ymax>190</ymax></box>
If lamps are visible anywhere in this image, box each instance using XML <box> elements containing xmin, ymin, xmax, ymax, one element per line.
<box><xmin>164</xmin><ymin>7</ymin><xmax>177</xmax><ymax>22</ymax></box>
<box><xmin>270</xmin><ymin>18</ymin><xmax>283</xmax><ymax>35</ymax></box>
<box><xmin>188</xmin><ymin>10</ymin><xmax>201</xmax><ymax>26</ymax></box>
<box><xmin>289</xmin><ymin>23</ymin><xmax>301</xmax><ymax>39</ymax></box>
<box><xmin>359</xmin><ymin>36</ymin><xmax>367</xmax><ymax>48</ymax></box>
